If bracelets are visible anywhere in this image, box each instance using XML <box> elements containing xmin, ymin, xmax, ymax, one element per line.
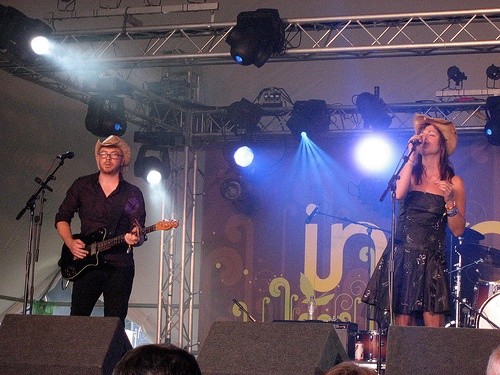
<box><xmin>409</xmin><ymin>159</ymin><xmax>415</xmax><ymax>164</ymax></box>
<box><xmin>445</xmin><ymin>209</ymin><xmax>458</xmax><ymax>217</ymax></box>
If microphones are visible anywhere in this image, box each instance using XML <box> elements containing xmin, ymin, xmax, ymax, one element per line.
<box><xmin>305</xmin><ymin>207</ymin><xmax>319</xmax><ymax>224</ymax></box>
<box><xmin>411</xmin><ymin>140</ymin><xmax>422</xmax><ymax>145</ymax></box>
<box><xmin>57</xmin><ymin>152</ymin><xmax>74</xmax><ymax>159</ymax></box>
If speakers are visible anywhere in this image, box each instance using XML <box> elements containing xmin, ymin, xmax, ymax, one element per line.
<box><xmin>385</xmin><ymin>325</ymin><xmax>500</xmax><ymax>375</ymax></box>
<box><xmin>0</xmin><ymin>314</ymin><xmax>133</xmax><ymax>375</ymax></box>
<box><xmin>196</xmin><ymin>320</ymin><xmax>359</xmax><ymax>375</ymax></box>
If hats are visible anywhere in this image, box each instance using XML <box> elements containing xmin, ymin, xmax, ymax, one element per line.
<box><xmin>94</xmin><ymin>135</ymin><xmax>131</xmax><ymax>171</ymax></box>
<box><xmin>413</xmin><ymin>114</ymin><xmax>457</xmax><ymax>156</ymax></box>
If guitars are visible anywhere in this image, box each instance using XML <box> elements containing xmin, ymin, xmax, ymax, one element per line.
<box><xmin>60</xmin><ymin>218</ymin><xmax>180</xmax><ymax>281</ymax></box>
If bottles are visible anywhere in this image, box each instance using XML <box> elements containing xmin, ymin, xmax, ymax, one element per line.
<box><xmin>354</xmin><ymin>341</ymin><xmax>364</xmax><ymax>361</ymax></box>
<box><xmin>307</xmin><ymin>297</ymin><xmax>318</xmax><ymax>320</ymax></box>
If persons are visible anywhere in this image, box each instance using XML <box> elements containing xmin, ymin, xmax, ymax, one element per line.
<box><xmin>362</xmin><ymin>115</ymin><xmax>467</xmax><ymax>328</ymax></box>
<box><xmin>486</xmin><ymin>345</ymin><xmax>500</xmax><ymax>375</ymax></box>
<box><xmin>113</xmin><ymin>344</ymin><xmax>201</xmax><ymax>375</ymax></box>
<box><xmin>327</xmin><ymin>360</ymin><xmax>377</xmax><ymax>375</ymax></box>
<box><xmin>55</xmin><ymin>135</ymin><xmax>147</xmax><ymax>329</ymax></box>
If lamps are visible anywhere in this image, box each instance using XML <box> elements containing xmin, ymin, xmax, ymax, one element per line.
<box><xmin>219</xmin><ymin>90</ymin><xmax>394</xmax><ymax>199</ymax></box>
<box><xmin>447</xmin><ymin>64</ymin><xmax>500</xmax><ymax>147</ymax></box>
<box><xmin>0</xmin><ymin>3</ymin><xmax>54</xmax><ymax>63</ymax></box>
<box><xmin>225</xmin><ymin>8</ymin><xmax>287</xmax><ymax>68</ymax></box>
<box><xmin>84</xmin><ymin>94</ymin><xmax>185</xmax><ymax>184</ymax></box>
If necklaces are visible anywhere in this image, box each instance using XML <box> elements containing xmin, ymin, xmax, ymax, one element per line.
<box><xmin>423</xmin><ymin>169</ymin><xmax>440</xmax><ymax>182</ymax></box>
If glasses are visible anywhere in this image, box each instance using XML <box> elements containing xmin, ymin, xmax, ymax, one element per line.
<box><xmin>99</xmin><ymin>151</ymin><xmax>123</xmax><ymax>159</ymax></box>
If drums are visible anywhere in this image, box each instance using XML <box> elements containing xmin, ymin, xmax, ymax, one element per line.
<box><xmin>356</xmin><ymin>331</ymin><xmax>389</xmax><ymax>362</ymax></box>
<box><xmin>467</xmin><ymin>276</ymin><xmax>500</xmax><ymax>329</ymax></box>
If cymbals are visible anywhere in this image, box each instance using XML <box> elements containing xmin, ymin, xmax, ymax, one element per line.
<box><xmin>456</xmin><ymin>243</ymin><xmax>500</xmax><ymax>268</ymax></box>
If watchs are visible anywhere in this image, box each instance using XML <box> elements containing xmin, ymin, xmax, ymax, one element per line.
<box><xmin>445</xmin><ymin>201</ymin><xmax>456</xmax><ymax>210</ymax></box>
